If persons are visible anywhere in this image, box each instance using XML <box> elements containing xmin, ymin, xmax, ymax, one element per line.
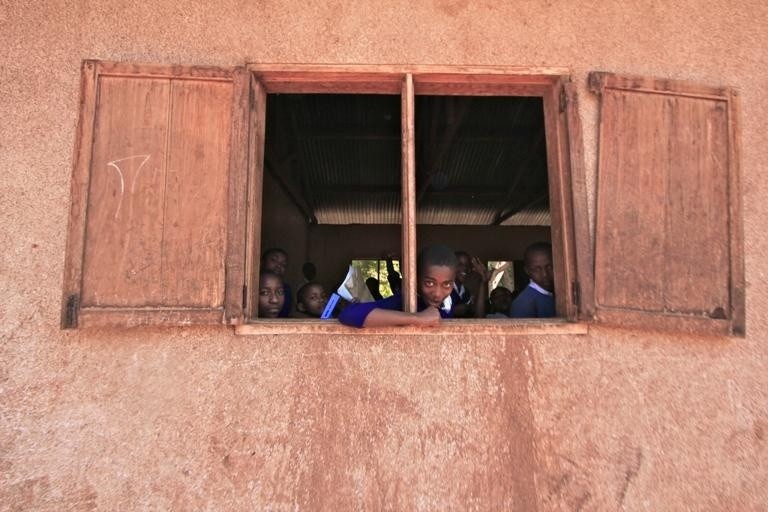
<box><xmin>258</xmin><ymin>242</ymin><xmax>557</xmax><ymax>319</ymax></box>
<box><xmin>338</xmin><ymin>245</ymin><xmax>460</xmax><ymax>328</ymax></box>
